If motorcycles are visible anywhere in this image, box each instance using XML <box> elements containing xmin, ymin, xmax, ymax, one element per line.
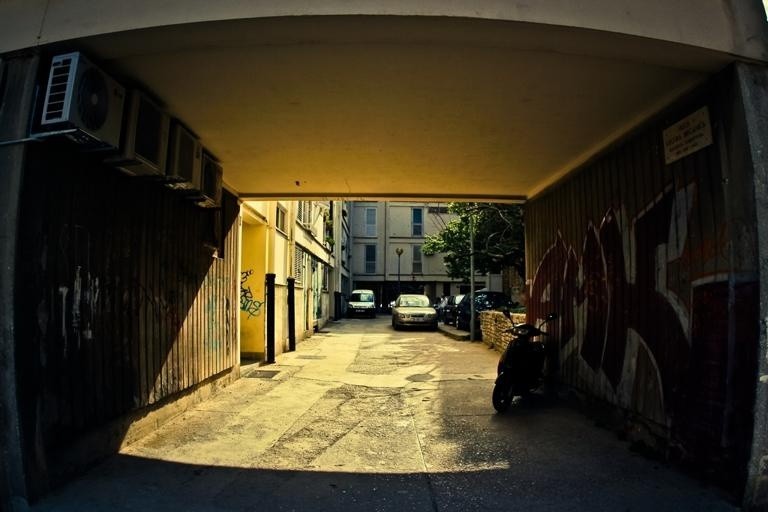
<box><xmin>491</xmin><ymin>307</ymin><xmax>557</xmax><ymax>413</ymax></box>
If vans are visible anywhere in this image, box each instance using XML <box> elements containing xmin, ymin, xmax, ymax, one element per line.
<box><xmin>347</xmin><ymin>289</ymin><xmax>376</xmax><ymax>318</ymax></box>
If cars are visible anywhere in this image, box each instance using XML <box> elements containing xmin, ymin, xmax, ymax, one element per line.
<box><xmin>433</xmin><ymin>288</ymin><xmax>520</xmax><ymax>331</ymax></box>
<box><xmin>391</xmin><ymin>293</ymin><xmax>439</xmax><ymax>331</ymax></box>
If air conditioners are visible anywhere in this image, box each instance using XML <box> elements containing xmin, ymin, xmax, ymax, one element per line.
<box><xmin>39</xmin><ymin>49</ymin><xmax>224</xmax><ymax>212</ymax></box>
